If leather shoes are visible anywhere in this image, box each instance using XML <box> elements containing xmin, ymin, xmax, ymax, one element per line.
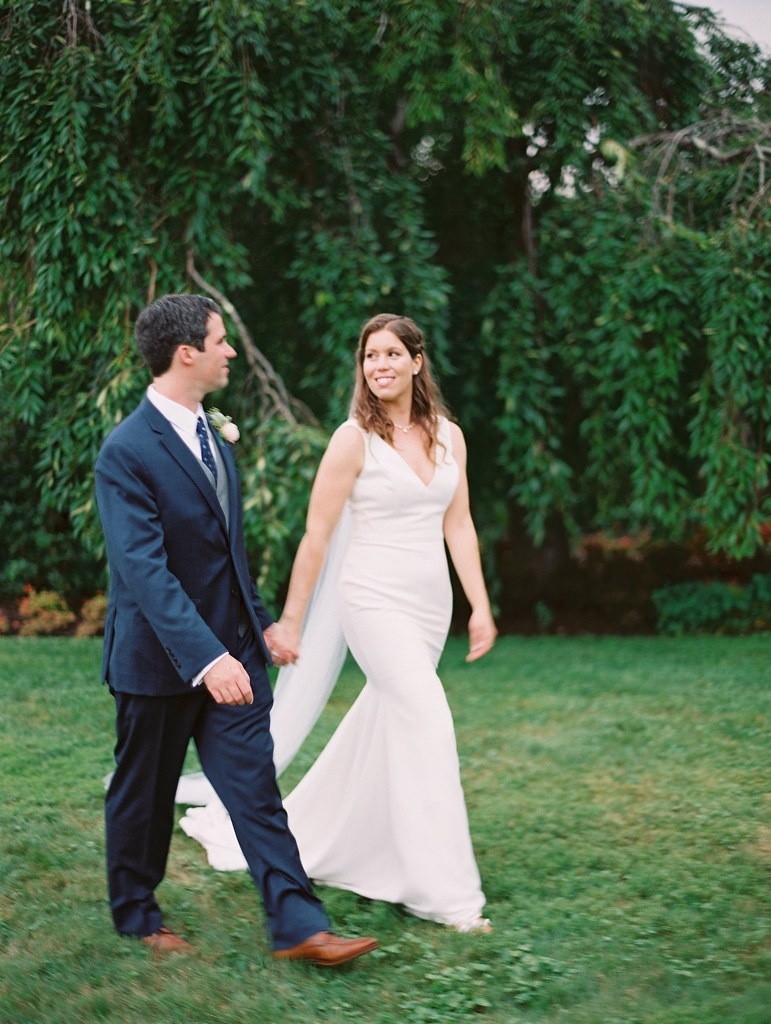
<box><xmin>271</xmin><ymin>931</ymin><xmax>379</xmax><ymax>966</ymax></box>
<box><xmin>141</xmin><ymin>924</ymin><xmax>192</xmax><ymax>954</ymax></box>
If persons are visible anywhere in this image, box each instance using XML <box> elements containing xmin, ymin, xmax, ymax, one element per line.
<box><xmin>93</xmin><ymin>294</ymin><xmax>378</xmax><ymax>967</ymax></box>
<box><xmin>275</xmin><ymin>315</ymin><xmax>496</xmax><ymax>934</ymax></box>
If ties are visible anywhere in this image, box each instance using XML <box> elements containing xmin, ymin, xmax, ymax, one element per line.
<box><xmin>197</xmin><ymin>416</ymin><xmax>218</xmax><ymax>487</ymax></box>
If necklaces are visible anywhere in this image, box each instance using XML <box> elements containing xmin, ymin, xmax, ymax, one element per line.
<box><xmin>390</xmin><ymin>420</ymin><xmax>416</xmax><ymax>433</ymax></box>
